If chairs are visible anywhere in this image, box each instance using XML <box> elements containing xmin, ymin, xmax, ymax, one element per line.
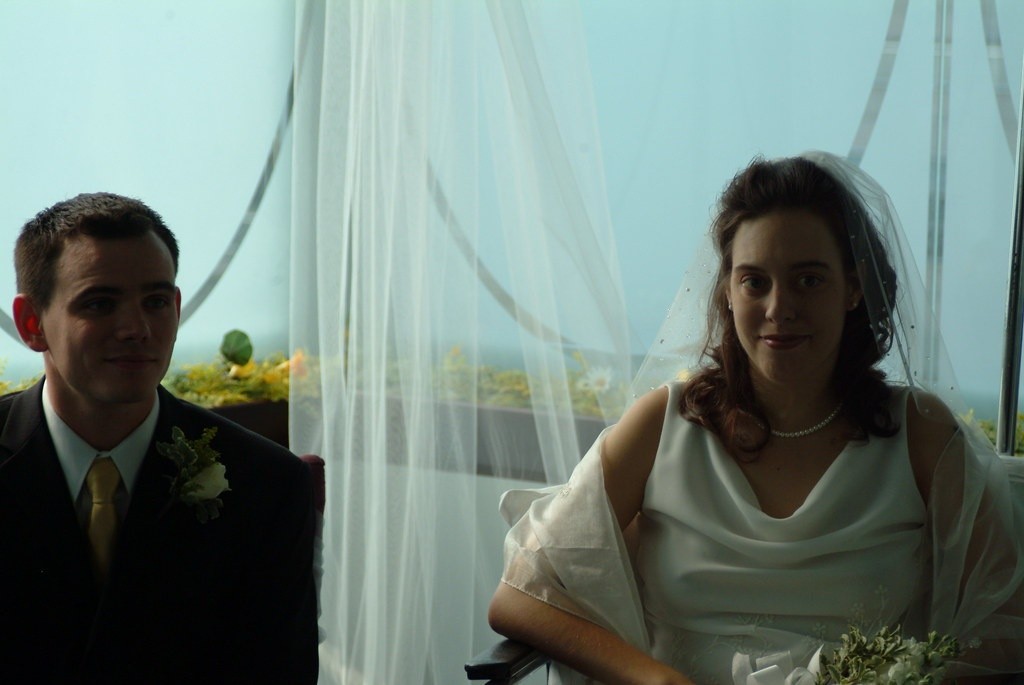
<box><xmin>464</xmin><ymin>455</ymin><xmax>1024</xmax><ymax>684</ymax></box>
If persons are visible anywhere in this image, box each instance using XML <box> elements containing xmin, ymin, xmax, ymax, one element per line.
<box><xmin>487</xmin><ymin>151</ymin><xmax>1024</xmax><ymax>685</ymax></box>
<box><xmin>0</xmin><ymin>192</ymin><xmax>322</xmax><ymax>685</ymax></box>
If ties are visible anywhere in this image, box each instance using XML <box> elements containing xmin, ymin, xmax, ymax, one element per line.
<box><xmin>84</xmin><ymin>456</ymin><xmax>121</xmax><ymax>584</ymax></box>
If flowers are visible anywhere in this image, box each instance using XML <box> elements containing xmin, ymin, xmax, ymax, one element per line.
<box><xmin>813</xmin><ymin>624</ymin><xmax>966</xmax><ymax>685</ymax></box>
<box><xmin>161</xmin><ymin>345</ymin><xmax>637</xmax><ymax>422</ymax></box>
<box><xmin>158</xmin><ymin>423</ymin><xmax>233</xmax><ymax>523</ymax></box>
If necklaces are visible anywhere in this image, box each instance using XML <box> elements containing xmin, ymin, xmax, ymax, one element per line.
<box><xmin>736</xmin><ymin>409</ymin><xmax>836</xmax><ymax>438</ymax></box>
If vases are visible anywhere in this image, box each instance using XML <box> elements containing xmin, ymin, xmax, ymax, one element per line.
<box><xmin>203</xmin><ymin>396</ymin><xmax>608</xmax><ymax>487</ymax></box>
